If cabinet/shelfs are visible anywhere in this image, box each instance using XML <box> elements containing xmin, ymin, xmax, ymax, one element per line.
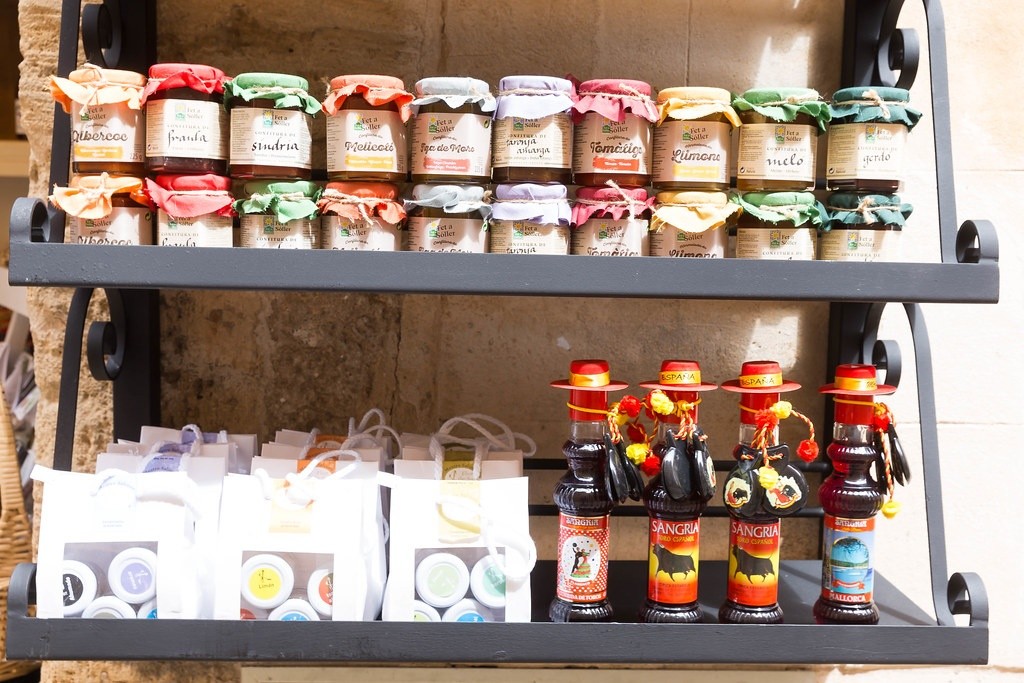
<box><xmin>4</xmin><ymin>0</ymin><xmax>999</xmax><ymax>667</ymax></box>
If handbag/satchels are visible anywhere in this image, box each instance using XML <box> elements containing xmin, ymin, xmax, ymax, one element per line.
<box><xmin>29</xmin><ymin>408</ymin><xmax>536</xmax><ymax>623</ymax></box>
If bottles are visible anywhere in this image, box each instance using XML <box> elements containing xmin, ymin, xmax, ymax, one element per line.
<box><xmin>548</xmin><ymin>360</ymin><xmax>615</xmax><ymax>622</ymax></box>
<box><xmin>645</xmin><ymin>361</ymin><xmax>706</xmax><ymax>624</ymax></box>
<box><xmin>814</xmin><ymin>364</ymin><xmax>887</xmax><ymax>622</ymax></box>
<box><xmin>720</xmin><ymin>362</ymin><xmax>786</xmax><ymax>625</ymax></box>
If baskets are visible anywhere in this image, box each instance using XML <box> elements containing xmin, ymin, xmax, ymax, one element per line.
<box><xmin>0</xmin><ymin>380</ymin><xmax>42</xmax><ymax>682</ymax></box>
